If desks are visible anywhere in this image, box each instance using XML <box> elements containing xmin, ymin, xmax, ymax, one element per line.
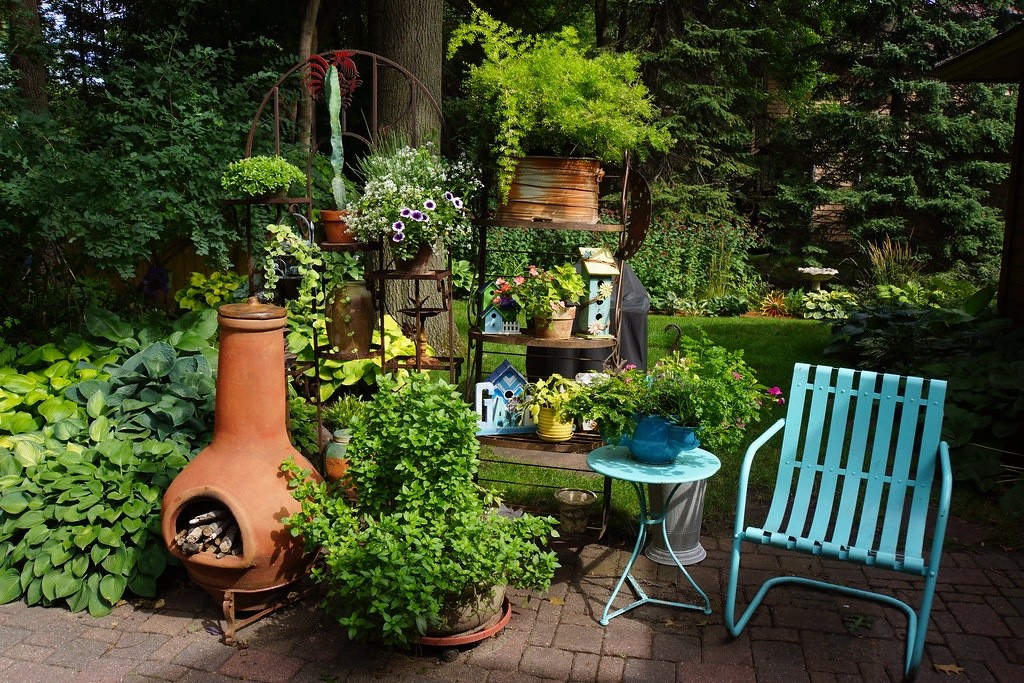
<box><xmin>584</xmin><ymin>447</ymin><xmax>721</xmax><ymax>625</ymax></box>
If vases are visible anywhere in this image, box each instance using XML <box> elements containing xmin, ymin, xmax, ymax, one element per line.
<box><xmin>387</xmin><ymin>234</ymin><xmax>432</xmax><ymax>274</ymax></box>
<box><xmin>531</xmin><ymin>305</ymin><xmax>575</xmax><ymax>340</ymax></box>
<box><xmin>613</xmin><ymin>413</ymin><xmax>701</xmax><ymax>462</ymax></box>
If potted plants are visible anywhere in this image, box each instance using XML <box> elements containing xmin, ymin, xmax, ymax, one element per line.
<box><xmin>439</xmin><ymin>7</ymin><xmax>661</xmax><ymax>224</ymax></box>
<box><xmin>320</xmin><ymin>68</ymin><xmax>362</xmax><ymax>242</ymax></box>
<box><xmin>301</xmin><ymin>249</ymin><xmax>376</xmax><ymax>357</ymax></box>
<box><xmin>517</xmin><ymin>373</ymin><xmax>586</xmax><ymax>444</ymax></box>
<box><xmin>272</xmin><ymin>457</ymin><xmax>562</xmax><ymax>652</ymax></box>
<box><xmin>259</xmin><ymin>223</ymin><xmax>318</xmax><ymax>307</ymax></box>
<box><xmin>222</xmin><ymin>157</ymin><xmax>306</xmax><ymax>200</ymax></box>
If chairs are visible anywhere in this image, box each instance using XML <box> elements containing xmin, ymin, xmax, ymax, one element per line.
<box><xmin>724</xmin><ymin>361</ymin><xmax>954</xmax><ymax>683</ymax></box>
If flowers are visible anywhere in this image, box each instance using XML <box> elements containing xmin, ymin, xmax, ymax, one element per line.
<box><xmin>344</xmin><ymin>128</ymin><xmax>485</xmax><ymax>264</ymax></box>
<box><xmin>596</xmin><ymin>356</ymin><xmax>785</xmax><ymax>456</ymax></box>
<box><xmin>494</xmin><ymin>265</ymin><xmax>588</xmax><ymax>332</ymax></box>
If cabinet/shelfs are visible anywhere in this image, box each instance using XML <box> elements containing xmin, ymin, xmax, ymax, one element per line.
<box><xmin>459</xmin><ymin>142</ymin><xmax>651</xmax><ymax>541</ymax></box>
